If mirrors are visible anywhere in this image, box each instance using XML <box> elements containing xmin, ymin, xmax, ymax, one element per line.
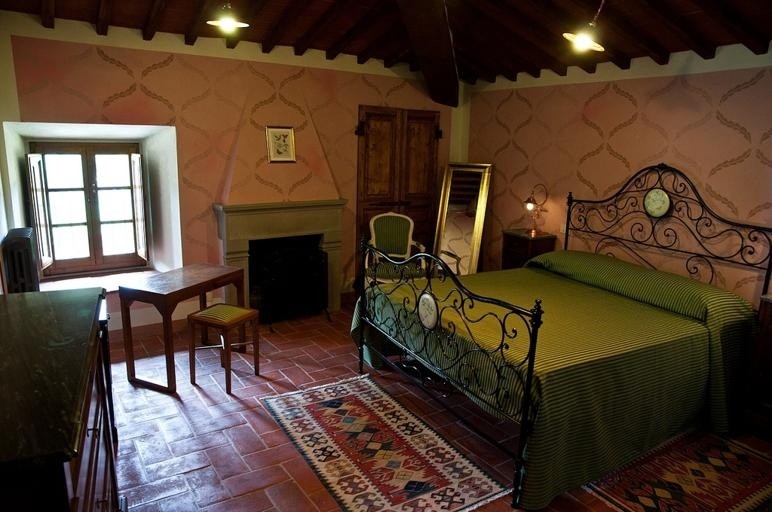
<box><xmin>428</xmin><ymin>160</ymin><xmax>492</xmax><ymax>275</ymax></box>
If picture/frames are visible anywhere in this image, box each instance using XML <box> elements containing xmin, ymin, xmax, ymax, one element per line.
<box><xmin>265</xmin><ymin>125</ymin><xmax>296</xmax><ymax>164</ymax></box>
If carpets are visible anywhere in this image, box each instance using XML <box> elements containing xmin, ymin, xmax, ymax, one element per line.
<box><xmin>579</xmin><ymin>416</ymin><xmax>772</xmax><ymax>511</ymax></box>
<box><xmin>259</xmin><ymin>366</ymin><xmax>513</xmax><ymax>510</ymax></box>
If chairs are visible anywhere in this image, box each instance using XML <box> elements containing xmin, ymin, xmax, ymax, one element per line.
<box><xmin>365</xmin><ymin>211</ymin><xmax>429</xmax><ymax>289</ymax></box>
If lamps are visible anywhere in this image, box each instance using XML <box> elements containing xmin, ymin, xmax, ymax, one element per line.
<box><xmin>562</xmin><ymin>0</ymin><xmax>615</xmax><ymax>56</ymax></box>
<box><xmin>525</xmin><ymin>182</ymin><xmax>550</xmax><ymax>238</ymax></box>
<box><xmin>206</xmin><ymin>0</ymin><xmax>251</xmax><ymax>34</ymax></box>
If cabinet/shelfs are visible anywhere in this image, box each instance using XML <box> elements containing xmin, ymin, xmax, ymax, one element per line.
<box><xmin>354</xmin><ymin>203</ymin><xmax>438</xmax><ymax>304</ymax></box>
<box><xmin>355</xmin><ymin>106</ymin><xmax>444</xmax><ymax>205</ymax></box>
<box><xmin>1</xmin><ymin>287</ymin><xmax>131</xmax><ymax>511</ymax></box>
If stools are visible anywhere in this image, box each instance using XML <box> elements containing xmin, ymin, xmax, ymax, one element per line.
<box><xmin>185</xmin><ymin>299</ymin><xmax>261</xmax><ymax>394</ymax></box>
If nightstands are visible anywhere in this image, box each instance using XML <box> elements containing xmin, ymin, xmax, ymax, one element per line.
<box><xmin>745</xmin><ymin>293</ymin><xmax>771</xmax><ymax>446</ymax></box>
<box><xmin>499</xmin><ymin>227</ymin><xmax>559</xmax><ymax>271</ymax></box>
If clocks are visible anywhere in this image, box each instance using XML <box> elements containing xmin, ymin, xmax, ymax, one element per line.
<box><xmin>640</xmin><ymin>187</ymin><xmax>670</xmax><ymax>219</ymax></box>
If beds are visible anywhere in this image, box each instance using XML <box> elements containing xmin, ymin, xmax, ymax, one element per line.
<box><xmin>356</xmin><ymin>162</ymin><xmax>771</xmax><ymax>511</ymax></box>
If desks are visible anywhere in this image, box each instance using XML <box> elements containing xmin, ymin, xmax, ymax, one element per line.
<box><xmin>119</xmin><ymin>263</ymin><xmax>250</xmax><ymax>395</ymax></box>
